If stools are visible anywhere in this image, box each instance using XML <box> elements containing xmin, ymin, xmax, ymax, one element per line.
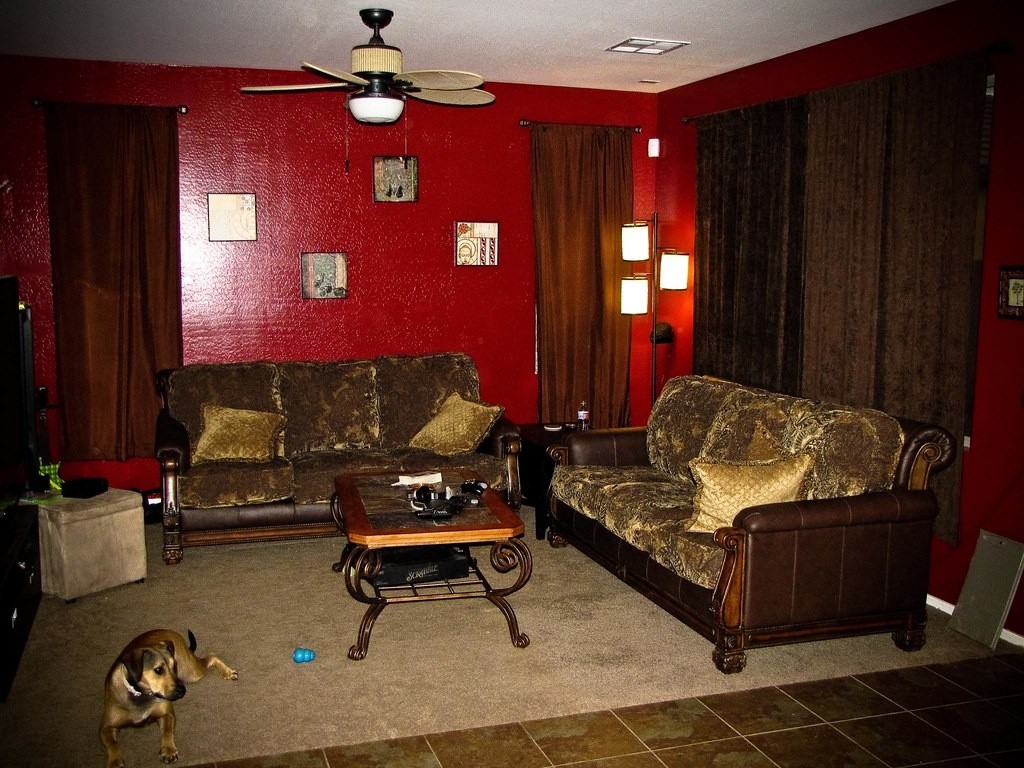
<box><xmin>17</xmin><ymin>488</ymin><xmax>148</xmax><ymax>604</ymax></box>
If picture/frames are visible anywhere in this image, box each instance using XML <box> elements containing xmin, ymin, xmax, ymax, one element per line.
<box><xmin>997</xmin><ymin>265</ymin><xmax>1024</xmax><ymax>322</ymax></box>
<box><xmin>455</xmin><ymin>221</ymin><xmax>499</xmax><ymax>266</ymax></box>
<box><xmin>371</xmin><ymin>154</ymin><xmax>419</xmax><ymax>203</ymax></box>
<box><xmin>207</xmin><ymin>193</ymin><xmax>257</xmax><ymax>241</ymax></box>
<box><xmin>300</xmin><ymin>252</ymin><xmax>348</xmax><ymax>298</ymax></box>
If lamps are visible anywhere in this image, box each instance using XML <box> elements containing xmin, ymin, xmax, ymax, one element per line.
<box><xmin>346</xmin><ymin>89</ymin><xmax>407</xmax><ymax>173</ymax></box>
<box><xmin>621</xmin><ymin>212</ymin><xmax>690</xmax><ymax>413</ymax></box>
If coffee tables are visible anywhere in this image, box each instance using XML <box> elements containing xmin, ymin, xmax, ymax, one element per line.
<box><xmin>331</xmin><ymin>468</ymin><xmax>535</xmax><ymax>661</ymax></box>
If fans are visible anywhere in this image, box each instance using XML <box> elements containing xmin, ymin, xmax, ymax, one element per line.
<box><xmin>240</xmin><ymin>7</ymin><xmax>496</xmax><ymax>106</ymax></box>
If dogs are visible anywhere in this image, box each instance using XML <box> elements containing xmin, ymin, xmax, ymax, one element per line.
<box><xmin>99</xmin><ymin>629</ymin><xmax>239</xmax><ymax>768</ymax></box>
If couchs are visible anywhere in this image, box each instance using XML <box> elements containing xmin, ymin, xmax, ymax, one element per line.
<box><xmin>542</xmin><ymin>373</ymin><xmax>958</xmax><ymax>675</ymax></box>
<box><xmin>154</xmin><ymin>352</ymin><xmax>523</xmax><ymax>566</ymax></box>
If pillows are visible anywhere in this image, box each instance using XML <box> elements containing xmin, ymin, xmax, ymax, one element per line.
<box><xmin>684</xmin><ymin>444</ymin><xmax>820</xmax><ymax>532</ymax></box>
<box><xmin>188</xmin><ymin>403</ymin><xmax>289</xmax><ymax>467</ymax></box>
<box><xmin>405</xmin><ymin>390</ymin><xmax>507</xmax><ymax>459</ymax></box>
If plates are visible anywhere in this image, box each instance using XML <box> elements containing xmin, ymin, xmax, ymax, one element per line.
<box><xmin>544</xmin><ymin>424</ymin><xmax>562</xmax><ymax>431</ymax></box>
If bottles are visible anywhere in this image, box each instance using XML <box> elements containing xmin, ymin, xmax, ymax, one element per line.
<box><xmin>578</xmin><ymin>400</ymin><xmax>590</xmax><ymax>431</ymax></box>
